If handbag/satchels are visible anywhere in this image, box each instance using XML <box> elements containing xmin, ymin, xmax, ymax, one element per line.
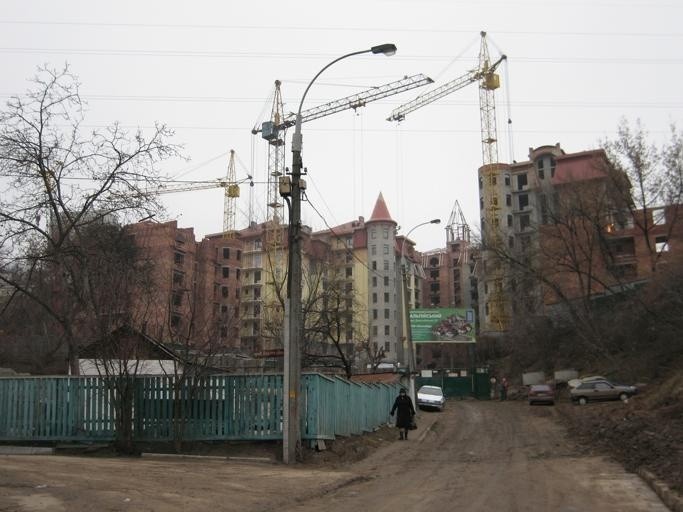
<box><xmin>409</xmin><ymin>422</ymin><xmax>417</xmax><ymax>430</ymax></box>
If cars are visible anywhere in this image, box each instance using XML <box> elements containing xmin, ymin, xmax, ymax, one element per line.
<box><xmin>416</xmin><ymin>385</ymin><xmax>445</xmax><ymax>411</ymax></box>
<box><xmin>526</xmin><ymin>376</ymin><xmax>637</xmax><ymax>406</ymax></box>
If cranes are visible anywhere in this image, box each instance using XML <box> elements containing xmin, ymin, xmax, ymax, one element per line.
<box><xmin>385</xmin><ymin>28</ymin><xmax>517</xmax><ymax>332</ymax></box>
<box><xmin>107</xmin><ymin>148</ymin><xmax>255</xmax><ymax>241</ymax></box>
<box><xmin>246</xmin><ymin>72</ymin><xmax>434</xmax><ymax>372</ymax></box>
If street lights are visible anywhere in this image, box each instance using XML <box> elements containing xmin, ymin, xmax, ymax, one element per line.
<box><xmin>282</xmin><ymin>42</ymin><xmax>399</xmax><ymax>463</ymax></box>
<box><xmin>400</xmin><ymin>218</ymin><xmax>442</xmax><ymax>412</ymax></box>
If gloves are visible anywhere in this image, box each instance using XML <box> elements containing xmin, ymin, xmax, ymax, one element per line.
<box><xmin>411</xmin><ymin>412</ymin><xmax>415</xmax><ymax>415</ymax></box>
<box><xmin>391</xmin><ymin>411</ymin><xmax>394</xmax><ymax>416</ymax></box>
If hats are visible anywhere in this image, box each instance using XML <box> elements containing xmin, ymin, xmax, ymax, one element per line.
<box><xmin>399</xmin><ymin>389</ymin><xmax>406</xmax><ymax>393</ymax></box>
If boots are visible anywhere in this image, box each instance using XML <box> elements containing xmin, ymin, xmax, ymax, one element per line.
<box><xmin>399</xmin><ymin>432</ymin><xmax>403</xmax><ymax>440</ymax></box>
<box><xmin>405</xmin><ymin>432</ymin><xmax>408</xmax><ymax>440</ymax></box>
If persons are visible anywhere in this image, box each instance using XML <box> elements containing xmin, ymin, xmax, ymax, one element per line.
<box><xmin>494</xmin><ymin>381</ymin><xmax>505</xmax><ymax>401</ymax></box>
<box><xmin>502</xmin><ymin>377</ymin><xmax>509</xmax><ymax>399</ymax></box>
<box><xmin>389</xmin><ymin>386</ymin><xmax>416</xmax><ymax>440</ymax></box>
